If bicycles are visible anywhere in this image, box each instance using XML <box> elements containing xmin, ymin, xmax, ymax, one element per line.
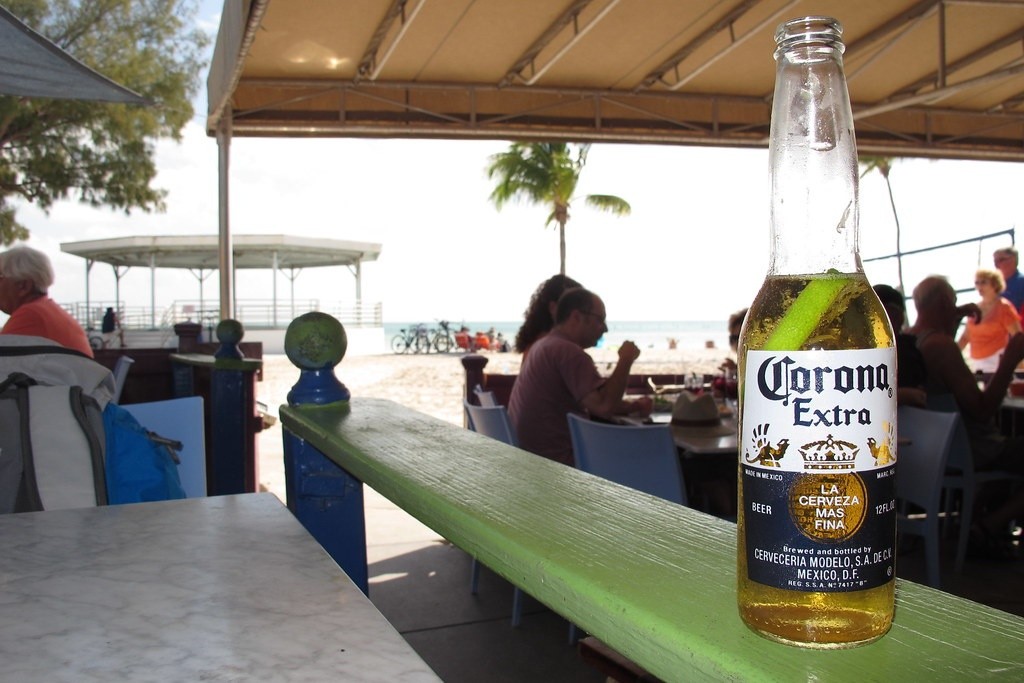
<box><xmin>391</xmin><ymin>321</ymin><xmax>453</xmax><ymax>354</ymax></box>
<box><xmin>84</xmin><ymin>327</ymin><xmax>104</xmax><ymax>349</ymax></box>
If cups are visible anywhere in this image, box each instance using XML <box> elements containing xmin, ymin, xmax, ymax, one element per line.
<box><xmin>684</xmin><ymin>374</ymin><xmax>704</xmax><ymax>397</ymax></box>
<box><xmin>983</xmin><ymin>372</ymin><xmax>998</xmax><ymax>392</ymax></box>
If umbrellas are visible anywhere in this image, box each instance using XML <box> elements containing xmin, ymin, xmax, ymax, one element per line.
<box><xmin>0</xmin><ymin>4</ymin><xmax>153</xmax><ymax>103</ymax></box>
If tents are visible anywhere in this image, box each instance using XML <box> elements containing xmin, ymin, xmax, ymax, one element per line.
<box><xmin>205</xmin><ymin>0</ymin><xmax>1024</xmax><ymax>317</ymax></box>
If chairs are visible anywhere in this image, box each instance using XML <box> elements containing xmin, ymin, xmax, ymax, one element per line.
<box><xmin>455</xmin><ymin>332</ymin><xmax>499</xmax><ymax>352</ymax></box>
<box><xmin>462</xmin><ymin>384</ymin><xmax>1024</xmax><ymax>647</ymax></box>
<box><xmin>111</xmin><ymin>352</ymin><xmax>137</xmax><ymax>405</ymax></box>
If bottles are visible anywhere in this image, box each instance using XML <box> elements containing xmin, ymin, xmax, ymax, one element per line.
<box><xmin>737</xmin><ymin>16</ymin><xmax>898</xmax><ymax>650</ymax></box>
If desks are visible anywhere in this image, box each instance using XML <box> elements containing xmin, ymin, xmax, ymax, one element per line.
<box><xmin>1</xmin><ymin>492</ymin><xmax>444</xmax><ymax>683</ymax></box>
<box><xmin>606</xmin><ymin>411</ymin><xmax>736</xmax><ymax>458</ymax></box>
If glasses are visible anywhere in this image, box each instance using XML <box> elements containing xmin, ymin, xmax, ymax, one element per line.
<box><xmin>582</xmin><ymin>311</ymin><xmax>605</xmax><ymax>323</ymax></box>
<box><xmin>729</xmin><ymin>335</ymin><xmax>739</xmax><ymax>343</ymax></box>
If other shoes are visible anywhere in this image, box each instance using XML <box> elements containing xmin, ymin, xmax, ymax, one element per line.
<box><xmin>970</xmin><ymin>520</ymin><xmax>1018</xmax><ymax>561</ymax></box>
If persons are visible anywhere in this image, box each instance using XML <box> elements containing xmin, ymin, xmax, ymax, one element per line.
<box><xmin>716</xmin><ymin>245</ymin><xmax>1024</xmax><ymax>562</ymax></box>
<box><xmin>506</xmin><ymin>273</ymin><xmax>654</xmax><ymax>467</ymax></box>
<box><xmin>102</xmin><ymin>307</ymin><xmax>127</xmax><ymax>347</ymax></box>
<box><xmin>462</xmin><ymin>326</ymin><xmax>505</xmax><ymax>352</ymax></box>
<box><xmin>0</xmin><ymin>245</ymin><xmax>94</xmax><ymax>357</ymax></box>
